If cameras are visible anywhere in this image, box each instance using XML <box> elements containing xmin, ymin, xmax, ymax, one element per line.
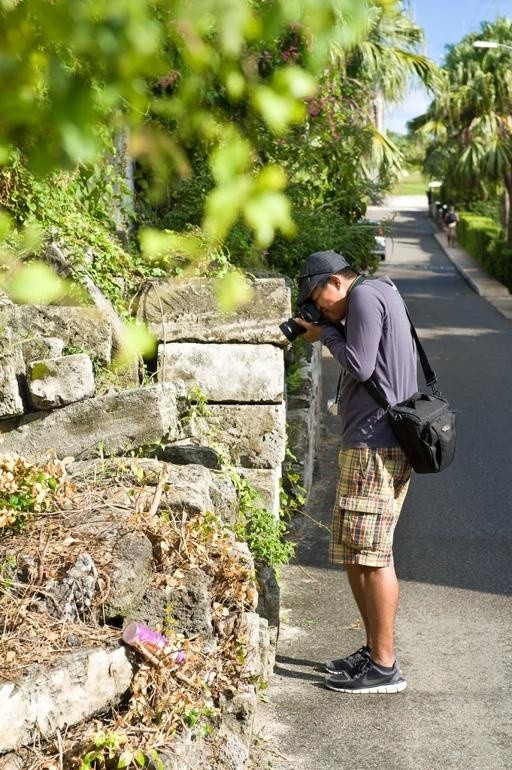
<box><xmin>279</xmin><ymin>303</ymin><xmax>322</xmax><ymax>342</ymax></box>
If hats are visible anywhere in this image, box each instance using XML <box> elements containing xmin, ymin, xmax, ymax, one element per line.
<box><xmin>297</xmin><ymin>251</ymin><xmax>351</xmax><ymax>307</ymax></box>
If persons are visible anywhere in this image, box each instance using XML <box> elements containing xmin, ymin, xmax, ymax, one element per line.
<box><xmin>295</xmin><ymin>250</ymin><xmax>422</xmax><ymax>695</ymax></box>
<box><xmin>444</xmin><ymin>205</ymin><xmax>459</xmax><ymax>247</ymax></box>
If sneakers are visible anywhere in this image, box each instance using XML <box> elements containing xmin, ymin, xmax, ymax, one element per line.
<box><xmin>323</xmin><ymin>646</ymin><xmax>408</xmax><ymax>694</ymax></box>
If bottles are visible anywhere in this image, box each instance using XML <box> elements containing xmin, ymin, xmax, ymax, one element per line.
<box><xmin>121</xmin><ymin>620</ymin><xmax>186</xmax><ymax>670</ymax></box>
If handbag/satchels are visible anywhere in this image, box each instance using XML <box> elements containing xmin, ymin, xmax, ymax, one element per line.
<box><xmin>389</xmin><ymin>392</ymin><xmax>456</xmax><ymax>473</ymax></box>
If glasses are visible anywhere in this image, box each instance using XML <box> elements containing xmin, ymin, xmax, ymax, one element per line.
<box><xmin>297</xmin><ymin>272</ymin><xmax>331</xmax><ymax>288</ymax></box>
